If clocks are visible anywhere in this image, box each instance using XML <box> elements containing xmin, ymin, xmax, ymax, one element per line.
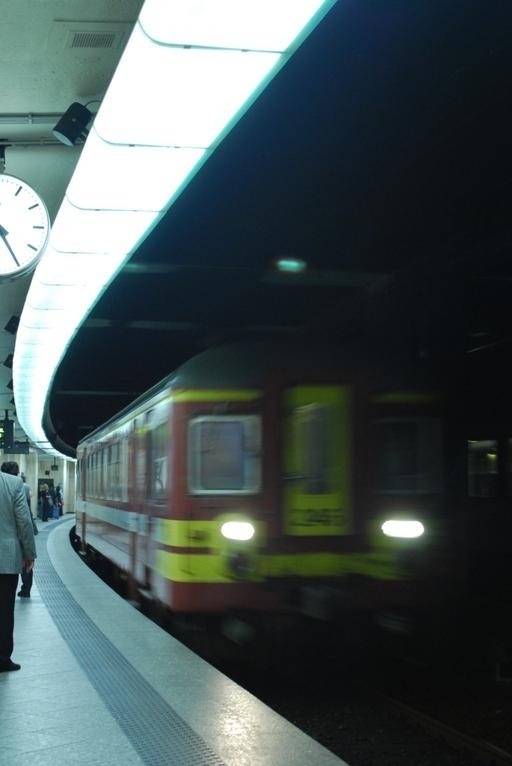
<box><xmin>0</xmin><ymin>144</ymin><xmax>51</xmax><ymax>284</ymax></box>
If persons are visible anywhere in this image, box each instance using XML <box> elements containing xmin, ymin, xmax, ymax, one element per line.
<box><xmin>20</xmin><ymin>476</ymin><xmax>65</xmax><ymax>536</ymax></box>
<box><xmin>0</xmin><ymin>462</ymin><xmax>37</xmax><ymax>674</ymax></box>
<box><xmin>0</xmin><ymin>461</ymin><xmax>38</xmax><ymax>598</ymax></box>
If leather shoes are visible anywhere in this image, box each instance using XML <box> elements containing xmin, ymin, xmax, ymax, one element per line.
<box><xmin>0</xmin><ymin>659</ymin><xmax>21</xmax><ymax>672</ymax></box>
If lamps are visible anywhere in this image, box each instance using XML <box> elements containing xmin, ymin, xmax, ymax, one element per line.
<box><xmin>3</xmin><ymin>353</ymin><xmax>17</xmax><ymax>418</ymax></box>
<box><xmin>53</xmin><ymin>102</ymin><xmax>89</xmax><ymax>149</ymax></box>
<box><xmin>4</xmin><ymin>314</ymin><xmax>19</xmax><ymax>335</ymax></box>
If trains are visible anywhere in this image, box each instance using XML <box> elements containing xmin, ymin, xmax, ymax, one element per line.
<box><xmin>75</xmin><ymin>335</ymin><xmax>441</xmax><ymax>645</ymax></box>
